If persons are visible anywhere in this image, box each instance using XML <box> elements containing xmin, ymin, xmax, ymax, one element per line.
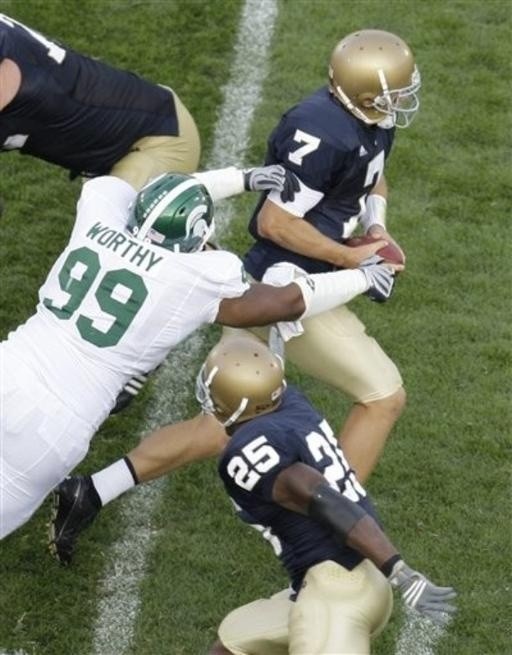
<box><xmin>0</xmin><ymin>160</ymin><xmax>405</xmax><ymax>544</ymax></box>
<box><xmin>44</xmin><ymin>27</ymin><xmax>421</xmax><ymax>567</ymax></box>
<box><xmin>0</xmin><ymin>7</ymin><xmax>203</xmax><ymax>419</ymax></box>
<box><xmin>195</xmin><ymin>332</ymin><xmax>459</xmax><ymax>655</ymax></box>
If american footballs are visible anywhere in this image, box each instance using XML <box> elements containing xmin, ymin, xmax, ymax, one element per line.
<box><xmin>333</xmin><ymin>233</ymin><xmax>405</xmax><ymax>278</ymax></box>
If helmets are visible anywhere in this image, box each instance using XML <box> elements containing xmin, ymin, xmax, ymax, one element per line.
<box><xmin>135</xmin><ymin>168</ymin><xmax>212</xmax><ymax>251</ymax></box>
<box><xmin>195</xmin><ymin>337</ymin><xmax>289</xmax><ymax>424</ymax></box>
<box><xmin>329</xmin><ymin>26</ymin><xmax>422</xmax><ymax>127</ymax></box>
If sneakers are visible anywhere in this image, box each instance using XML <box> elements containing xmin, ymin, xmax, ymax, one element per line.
<box><xmin>47</xmin><ymin>476</ymin><xmax>89</xmax><ymax>570</ymax></box>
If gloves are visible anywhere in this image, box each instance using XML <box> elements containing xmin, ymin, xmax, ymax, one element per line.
<box><xmin>364</xmin><ymin>263</ymin><xmax>399</xmax><ymax>306</ymax></box>
<box><xmin>244</xmin><ymin>158</ymin><xmax>301</xmax><ymax>205</ymax></box>
<box><xmin>391</xmin><ymin>565</ymin><xmax>457</xmax><ymax>626</ymax></box>
